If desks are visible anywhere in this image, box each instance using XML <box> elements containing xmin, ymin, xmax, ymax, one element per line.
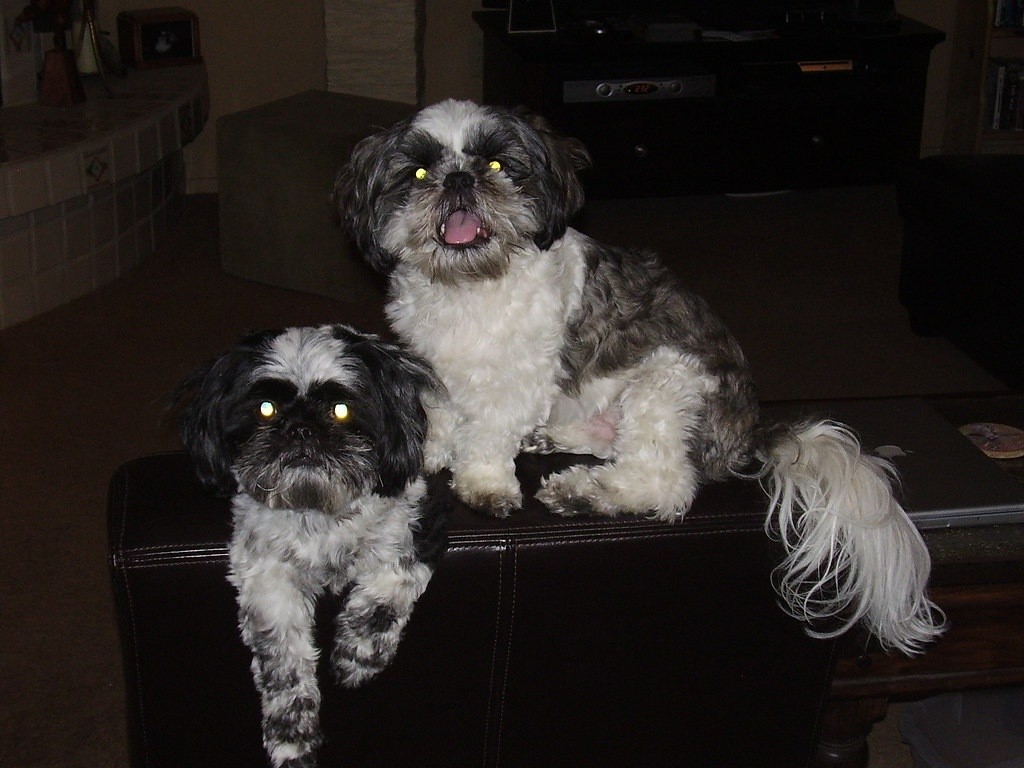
<box><xmin>750</xmin><ymin>392</ymin><xmax>1024</xmax><ymax>768</ymax></box>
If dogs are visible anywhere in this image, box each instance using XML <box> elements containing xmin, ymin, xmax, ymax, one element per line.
<box><xmin>149</xmin><ymin>321</ymin><xmax>454</xmax><ymax>768</ymax></box>
<box><xmin>331</xmin><ymin>96</ymin><xmax>951</xmax><ymax>662</ymax></box>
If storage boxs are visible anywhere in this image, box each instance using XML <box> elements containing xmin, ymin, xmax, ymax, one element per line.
<box><xmin>117</xmin><ymin>6</ymin><xmax>204</xmax><ymax>69</ymax></box>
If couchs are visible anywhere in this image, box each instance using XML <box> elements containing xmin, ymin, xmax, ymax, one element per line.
<box><xmin>895</xmin><ymin>153</ymin><xmax>1024</xmax><ymax>398</ymax></box>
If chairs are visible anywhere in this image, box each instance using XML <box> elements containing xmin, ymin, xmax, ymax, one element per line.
<box><xmin>105</xmin><ymin>450</ymin><xmax>824</xmax><ymax>768</ymax></box>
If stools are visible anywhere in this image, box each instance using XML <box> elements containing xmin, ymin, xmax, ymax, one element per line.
<box><xmin>215</xmin><ymin>88</ymin><xmax>413</xmax><ymax>310</ymax></box>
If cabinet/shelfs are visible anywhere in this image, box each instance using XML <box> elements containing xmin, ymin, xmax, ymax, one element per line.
<box><xmin>474</xmin><ymin>10</ymin><xmax>947</xmax><ymax>197</ymax></box>
<box><xmin>942</xmin><ymin>0</ymin><xmax>1024</xmax><ymax>153</ymax></box>
